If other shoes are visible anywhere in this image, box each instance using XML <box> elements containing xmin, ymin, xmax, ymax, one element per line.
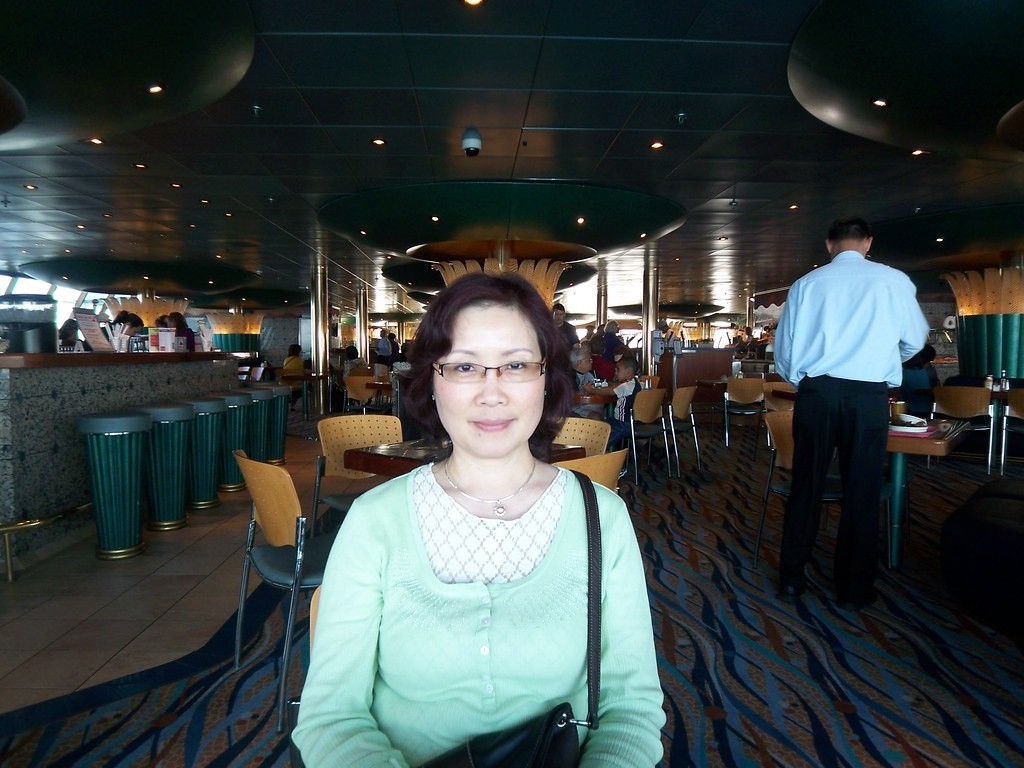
<box><xmin>780</xmin><ymin>584</ymin><xmax>800</xmax><ymax>606</ymax></box>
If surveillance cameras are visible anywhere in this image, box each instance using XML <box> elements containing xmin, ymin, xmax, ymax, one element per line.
<box><xmin>462</xmin><ymin>137</ymin><xmax>482</xmax><ymax>157</ymax></box>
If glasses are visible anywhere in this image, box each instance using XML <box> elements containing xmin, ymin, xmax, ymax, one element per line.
<box><xmin>431</xmin><ymin>359</ymin><xmax>549</xmax><ymax>385</ymax></box>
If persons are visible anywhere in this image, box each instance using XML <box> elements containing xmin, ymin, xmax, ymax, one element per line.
<box><xmin>660</xmin><ymin>322</ymin><xmax>679</xmax><ymax>347</ymax></box>
<box><xmin>284</xmin><ymin>329</ymin><xmax>399</xmax><ymax>410</ymax></box>
<box><xmin>293</xmin><ymin>272</ymin><xmax>667</xmax><ymax>768</ymax></box>
<box><xmin>773</xmin><ymin>217</ymin><xmax>929</xmax><ymax>609</ymax></box>
<box><xmin>552</xmin><ymin>303</ymin><xmax>641</xmax><ymax>480</ymax></box>
<box><xmin>59</xmin><ymin>310</ymin><xmax>195</xmax><ymax>351</ymax></box>
<box><xmin>894</xmin><ymin>344</ymin><xmax>941</xmax><ymax>419</ymax></box>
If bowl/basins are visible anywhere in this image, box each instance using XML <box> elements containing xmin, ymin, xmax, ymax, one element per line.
<box><xmin>894</xmin><ymin>412</ymin><xmax>927</xmax><ymax>426</ymax></box>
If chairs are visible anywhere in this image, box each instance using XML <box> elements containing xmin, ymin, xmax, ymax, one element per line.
<box><xmin>620</xmin><ymin>389</ymin><xmax>672</xmax><ymax>486</ymax></box>
<box><xmin>343</xmin><ymin>367</ymin><xmax>393</xmax><ymax>413</ymax></box>
<box><xmin>722</xmin><ymin>378</ymin><xmax>842</xmax><ymax>570</ymax></box>
<box><xmin>232</xmin><ymin>448</ymin><xmax>335</xmax><ymax>734</ymax></box>
<box><xmin>1000</xmin><ymin>388</ymin><xmax>1023</xmax><ymax>475</ymax></box>
<box><xmin>238</xmin><ymin>365</ymin><xmax>265</xmax><ymax>381</ymax></box>
<box><xmin>648</xmin><ymin>385</ymin><xmax>701</xmax><ymax>468</ymax></box>
<box><xmin>549</xmin><ymin>447</ymin><xmax>629</xmax><ymax>490</ymax></box>
<box><xmin>310</xmin><ymin>414</ymin><xmax>403</xmax><ymax>540</ymax></box>
<box><xmin>926</xmin><ymin>386</ymin><xmax>996</xmax><ymax>474</ymax></box>
<box><xmin>553</xmin><ymin>417</ymin><xmax>612</xmax><ymax>455</ymax></box>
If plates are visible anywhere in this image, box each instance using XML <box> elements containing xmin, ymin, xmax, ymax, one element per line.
<box><xmin>889</xmin><ymin>424</ymin><xmax>928</xmax><ymax>433</ymax></box>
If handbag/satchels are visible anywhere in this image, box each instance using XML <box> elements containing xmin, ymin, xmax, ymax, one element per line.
<box><xmin>418</xmin><ymin>701</ymin><xmax>582</xmax><ymax>768</ymax></box>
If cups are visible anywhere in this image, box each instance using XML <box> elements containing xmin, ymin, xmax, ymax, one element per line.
<box><xmin>148</xmin><ymin>328</ymin><xmax>159</xmax><ymax>351</ymax></box>
<box><xmin>158</xmin><ymin>328</ymin><xmax>177</xmax><ymax>352</ymax></box>
<box><xmin>130</xmin><ymin>337</ymin><xmax>145</xmax><ymax>352</ymax></box>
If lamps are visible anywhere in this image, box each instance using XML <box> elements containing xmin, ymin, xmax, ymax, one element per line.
<box><xmin>461</xmin><ymin>127</ymin><xmax>482</xmax><ymax>158</ymax></box>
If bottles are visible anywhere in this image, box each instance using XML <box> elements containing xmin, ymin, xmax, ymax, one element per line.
<box><xmin>984</xmin><ymin>374</ymin><xmax>993</xmax><ymax>391</ymax></box>
<box><xmin>1000</xmin><ymin>369</ymin><xmax>1010</xmax><ymax>391</ymax></box>
<box><xmin>735</xmin><ymin>370</ymin><xmax>744</xmax><ymax>379</ymax></box>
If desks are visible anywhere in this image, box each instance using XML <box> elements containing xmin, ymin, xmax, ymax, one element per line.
<box><xmin>365</xmin><ymin>382</ymin><xmax>392</xmax><ymax>398</ymax></box>
<box><xmin>344</xmin><ymin>436</ymin><xmax>586</xmax><ymax>475</ymax></box>
<box><xmin>883</xmin><ymin>420</ymin><xmax>971</xmax><ymax>567</ymax></box>
<box><xmin>277</xmin><ymin>369</ymin><xmax>313</xmax><ymax>380</ymax></box>
<box><xmin>570</xmin><ymin>381</ymin><xmax>630</xmax><ymax>406</ymax></box>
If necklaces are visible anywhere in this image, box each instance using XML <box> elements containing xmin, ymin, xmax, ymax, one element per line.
<box><xmin>444</xmin><ymin>457</ymin><xmax>535</xmax><ymax>517</ymax></box>
